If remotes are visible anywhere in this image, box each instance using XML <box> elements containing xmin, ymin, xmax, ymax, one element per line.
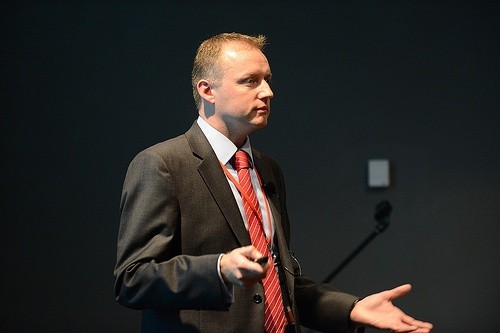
<box><xmin>256</xmin><ymin>256</ymin><xmax>269</xmax><ymax>265</ymax></box>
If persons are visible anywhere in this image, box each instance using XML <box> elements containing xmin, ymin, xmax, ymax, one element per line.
<box><xmin>113</xmin><ymin>34</ymin><xmax>433</xmax><ymax>333</ymax></box>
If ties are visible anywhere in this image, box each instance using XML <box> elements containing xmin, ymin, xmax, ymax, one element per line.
<box><xmin>230</xmin><ymin>150</ymin><xmax>288</xmax><ymax>333</ymax></box>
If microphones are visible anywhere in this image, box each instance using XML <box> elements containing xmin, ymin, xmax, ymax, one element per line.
<box><xmin>264</xmin><ymin>182</ymin><xmax>276</xmax><ymax>196</ymax></box>
